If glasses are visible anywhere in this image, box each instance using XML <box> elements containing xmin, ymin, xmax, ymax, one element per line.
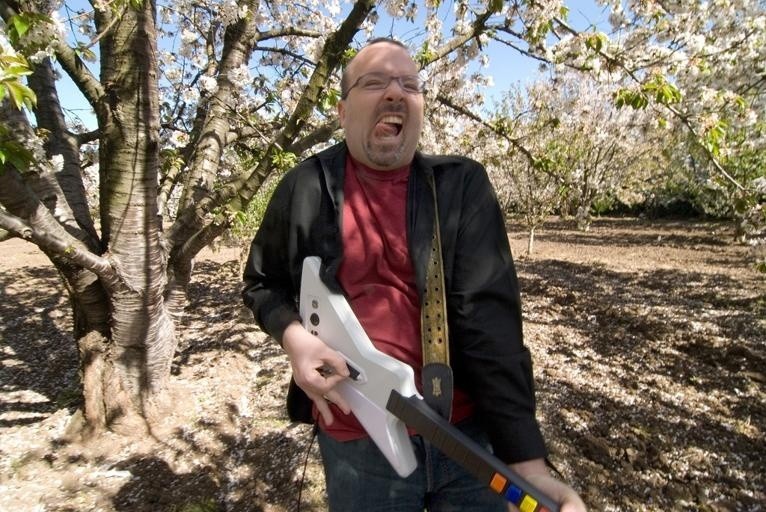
<box><xmin>345</xmin><ymin>70</ymin><xmax>425</xmax><ymax>93</ymax></box>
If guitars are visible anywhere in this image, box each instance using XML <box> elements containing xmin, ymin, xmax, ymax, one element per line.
<box><xmin>299</xmin><ymin>256</ymin><xmax>561</xmax><ymax>512</ymax></box>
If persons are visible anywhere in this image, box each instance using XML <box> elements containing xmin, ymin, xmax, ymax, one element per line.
<box><xmin>240</xmin><ymin>37</ymin><xmax>588</xmax><ymax>512</ymax></box>
